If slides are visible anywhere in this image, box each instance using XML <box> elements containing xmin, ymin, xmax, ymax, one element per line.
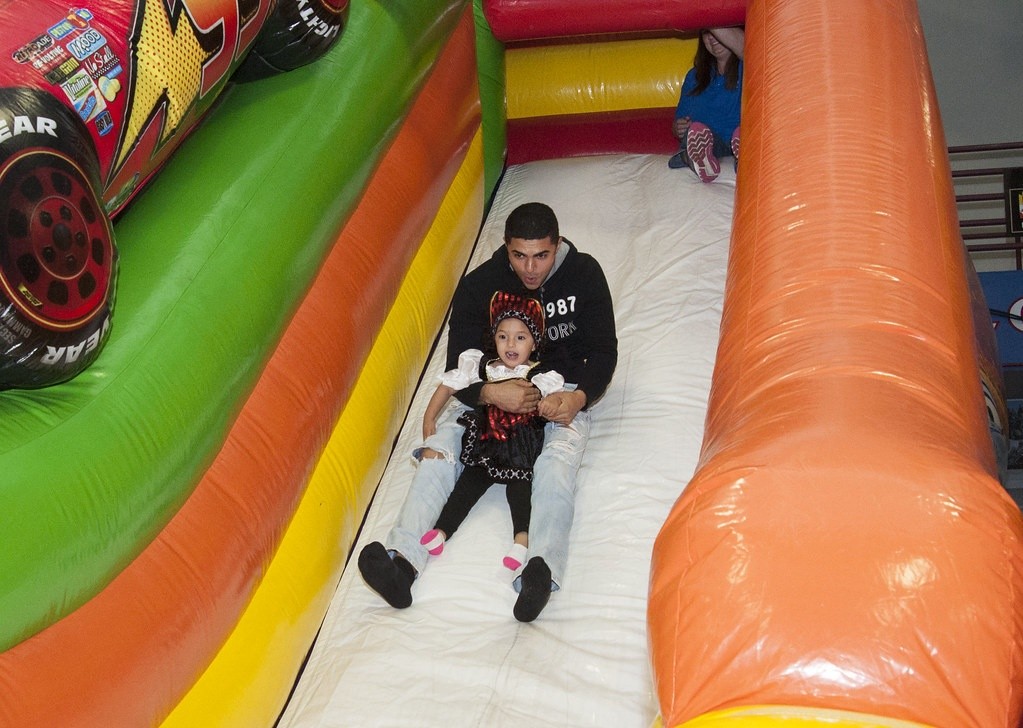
<box><xmin>275</xmin><ymin>148</ymin><xmax>745</xmax><ymax>728</ymax></box>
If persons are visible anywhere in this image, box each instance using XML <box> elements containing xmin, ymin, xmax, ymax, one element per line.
<box><xmin>668</xmin><ymin>29</ymin><xmax>745</xmax><ymax>183</ymax></box>
<box><xmin>421</xmin><ymin>289</ymin><xmax>566</xmax><ymax>569</ymax></box>
<box><xmin>358</xmin><ymin>201</ymin><xmax>618</xmax><ymax>623</ymax></box>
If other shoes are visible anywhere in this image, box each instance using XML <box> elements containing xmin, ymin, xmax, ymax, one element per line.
<box><xmin>684</xmin><ymin>121</ymin><xmax>720</xmax><ymax>183</ymax></box>
<box><xmin>729</xmin><ymin>127</ymin><xmax>739</xmax><ymax>173</ymax></box>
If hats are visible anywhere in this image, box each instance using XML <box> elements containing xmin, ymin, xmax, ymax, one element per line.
<box><xmin>488</xmin><ymin>291</ymin><xmax>546</xmax><ymax>348</ymax></box>
